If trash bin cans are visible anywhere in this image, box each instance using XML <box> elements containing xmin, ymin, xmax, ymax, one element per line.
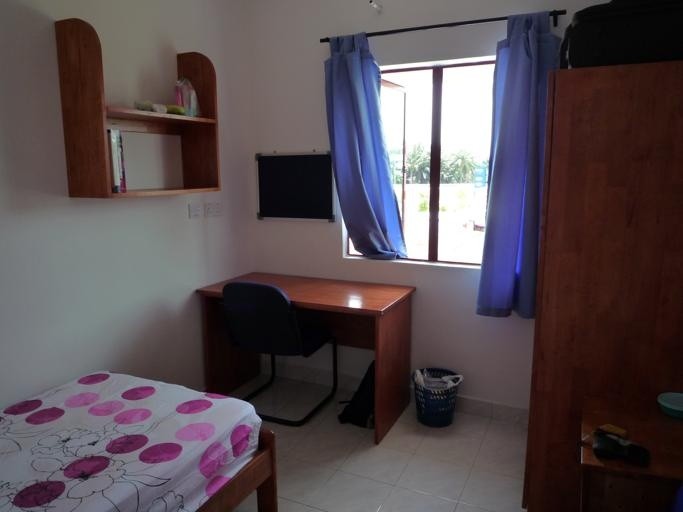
<box><xmin>411</xmin><ymin>367</ymin><xmax>464</xmax><ymax>427</ymax></box>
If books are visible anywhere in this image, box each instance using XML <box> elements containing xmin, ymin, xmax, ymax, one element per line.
<box><xmin>107</xmin><ymin>129</ymin><xmax>127</xmax><ymax>193</ymax></box>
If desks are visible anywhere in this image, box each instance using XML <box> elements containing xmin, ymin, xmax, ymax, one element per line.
<box><xmin>576</xmin><ymin>395</ymin><xmax>683</xmax><ymax>512</ymax></box>
<box><xmin>196</xmin><ymin>272</ymin><xmax>417</xmax><ymax>445</ymax></box>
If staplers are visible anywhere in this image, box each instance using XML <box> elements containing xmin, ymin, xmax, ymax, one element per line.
<box><xmin>590</xmin><ymin>428</ymin><xmax>650</xmax><ymax>468</ymax></box>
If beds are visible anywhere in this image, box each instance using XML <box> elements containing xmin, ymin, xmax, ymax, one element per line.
<box><xmin>0</xmin><ymin>369</ymin><xmax>278</xmax><ymax>512</ymax></box>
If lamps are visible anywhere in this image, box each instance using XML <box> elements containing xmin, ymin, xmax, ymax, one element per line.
<box><xmin>367</xmin><ymin>0</ymin><xmax>381</xmax><ymax>12</ymax></box>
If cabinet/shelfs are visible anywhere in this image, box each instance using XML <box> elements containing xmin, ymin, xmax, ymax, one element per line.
<box><xmin>54</xmin><ymin>18</ymin><xmax>221</xmax><ymax>198</ymax></box>
<box><xmin>522</xmin><ymin>62</ymin><xmax>683</xmax><ymax>512</ymax></box>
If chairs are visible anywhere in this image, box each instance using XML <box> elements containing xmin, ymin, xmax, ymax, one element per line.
<box><xmin>218</xmin><ymin>281</ymin><xmax>340</xmax><ymax>426</ymax></box>
